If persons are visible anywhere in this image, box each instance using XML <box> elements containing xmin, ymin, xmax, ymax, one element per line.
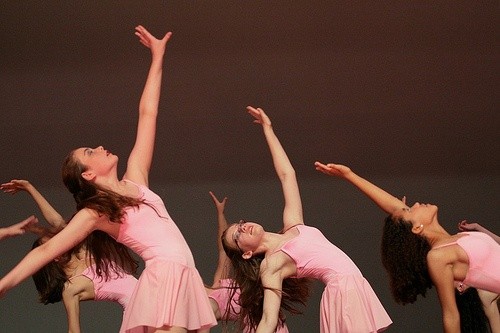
<box><xmin>1</xmin><ymin>177</ymin><xmax>500</xmax><ymax>333</ymax></box>
<box><xmin>315</xmin><ymin>161</ymin><xmax>500</xmax><ymax>333</ymax></box>
<box><xmin>223</xmin><ymin>108</ymin><xmax>392</xmax><ymax>332</ymax></box>
<box><xmin>1</xmin><ymin>25</ymin><xmax>216</xmax><ymax>332</ymax></box>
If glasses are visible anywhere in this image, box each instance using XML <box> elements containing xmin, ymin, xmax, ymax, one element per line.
<box><xmin>232</xmin><ymin>218</ymin><xmax>245</xmax><ymax>254</ymax></box>
